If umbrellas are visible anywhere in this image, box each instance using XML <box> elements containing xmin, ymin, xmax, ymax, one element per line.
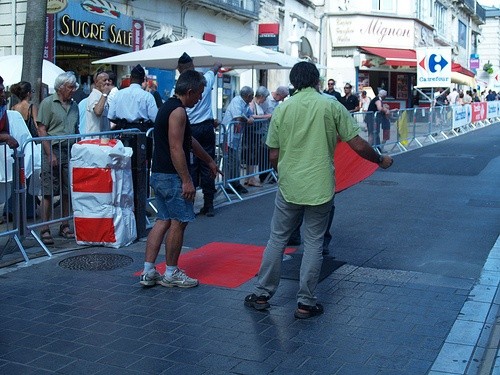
<box><xmin>221</xmin><ymin>44</ymin><xmax>330</xmax><ymax>89</ymax></box>
<box><xmin>0</xmin><ymin>56</ymin><xmax>78</xmax><ymax>94</ymax></box>
<box><xmin>92</xmin><ymin>36</ymin><xmax>279</xmax><ymax>69</ymax></box>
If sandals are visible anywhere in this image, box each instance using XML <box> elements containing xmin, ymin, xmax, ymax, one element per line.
<box><xmin>40</xmin><ymin>228</ymin><xmax>54</xmax><ymax>244</ymax></box>
<box><xmin>59</xmin><ymin>223</ymin><xmax>75</xmax><ymax>239</ymax></box>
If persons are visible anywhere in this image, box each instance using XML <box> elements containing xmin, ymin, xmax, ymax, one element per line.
<box><xmin>78</xmin><ymin>72</ymin><xmax>111</xmax><ymax>138</ymax></box>
<box><xmin>434</xmin><ymin>87</ymin><xmax>500</xmax><ymax>132</ymax></box>
<box><xmin>4</xmin><ymin>82</ymin><xmax>40</xmax><ymax>221</ymax></box>
<box><xmin>360</xmin><ymin>90</ymin><xmax>398</xmax><ymax>153</ymax></box>
<box><xmin>140</xmin><ymin>73</ymin><xmax>218</xmax><ymax>287</ymax></box>
<box><xmin>106</xmin><ymin>62</ymin><xmax>162</xmax><ymax>217</ymax></box>
<box><xmin>218</xmin><ymin>86</ymin><xmax>254</xmax><ymax>194</ymax></box>
<box><xmin>0</xmin><ymin>76</ymin><xmax>19</xmax><ymax>149</ymax></box>
<box><xmin>325</xmin><ymin>79</ymin><xmax>359</xmax><ymax>118</ymax></box>
<box><xmin>243</xmin><ymin>86</ymin><xmax>288</xmax><ymax>187</ymax></box>
<box><xmin>178</xmin><ymin>53</ymin><xmax>220</xmax><ymax>216</ymax></box>
<box><xmin>36</xmin><ymin>71</ymin><xmax>80</xmax><ymax>243</ymax></box>
<box><xmin>245</xmin><ymin>61</ymin><xmax>394</xmax><ymax>318</ymax></box>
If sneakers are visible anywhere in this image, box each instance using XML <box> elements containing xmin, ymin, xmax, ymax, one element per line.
<box><xmin>160</xmin><ymin>268</ymin><xmax>199</xmax><ymax>288</ymax></box>
<box><xmin>294</xmin><ymin>301</ymin><xmax>324</xmax><ymax>319</ymax></box>
<box><xmin>244</xmin><ymin>294</ymin><xmax>270</xmax><ymax>310</ymax></box>
<box><xmin>140</xmin><ymin>266</ymin><xmax>163</xmax><ymax>286</ymax></box>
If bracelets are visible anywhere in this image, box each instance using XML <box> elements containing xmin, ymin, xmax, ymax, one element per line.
<box><xmin>377</xmin><ymin>155</ymin><xmax>383</xmax><ymax>163</ymax></box>
<box><xmin>103</xmin><ymin>94</ymin><xmax>107</xmax><ymax>96</ymax></box>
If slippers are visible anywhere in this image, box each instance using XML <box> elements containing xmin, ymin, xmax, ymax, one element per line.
<box><xmin>244</xmin><ymin>181</ymin><xmax>263</xmax><ymax>186</ymax></box>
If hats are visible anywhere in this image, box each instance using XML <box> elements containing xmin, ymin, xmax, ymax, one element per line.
<box><xmin>177</xmin><ymin>52</ymin><xmax>193</xmax><ymax>64</ymax></box>
<box><xmin>131</xmin><ymin>64</ymin><xmax>145</xmax><ymax>78</ymax></box>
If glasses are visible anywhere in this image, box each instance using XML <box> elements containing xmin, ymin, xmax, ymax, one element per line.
<box><xmin>328</xmin><ymin>83</ymin><xmax>334</xmax><ymax>85</ymax></box>
<box><xmin>344</xmin><ymin>87</ymin><xmax>349</xmax><ymax>89</ymax></box>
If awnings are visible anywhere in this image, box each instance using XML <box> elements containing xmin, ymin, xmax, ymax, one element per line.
<box><xmin>360</xmin><ymin>46</ymin><xmax>417</xmax><ymax>66</ymax></box>
<box><xmin>452</xmin><ymin>63</ymin><xmax>475</xmax><ymax>86</ymax></box>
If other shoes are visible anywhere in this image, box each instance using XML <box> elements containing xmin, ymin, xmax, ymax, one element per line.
<box><xmin>259</xmin><ymin>176</ymin><xmax>278</xmax><ymax>184</ymax></box>
<box><xmin>137</xmin><ymin>226</ymin><xmax>154</xmax><ymax>240</ymax></box>
<box><xmin>205</xmin><ymin>206</ymin><xmax>214</xmax><ymax>217</ymax></box>
<box><xmin>323</xmin><ymin>242</ymin><xmax>329</xmax><ymax>255</ymax></box>
<box><xmin>286</xmin><ymin>239</ymin><xmax>301</xmax><ymax>246</ymax></box>
<box><xmin>226</xmin><ymin>183</ymin><xmax>248</xmax><ymax>194</ymax></box>
